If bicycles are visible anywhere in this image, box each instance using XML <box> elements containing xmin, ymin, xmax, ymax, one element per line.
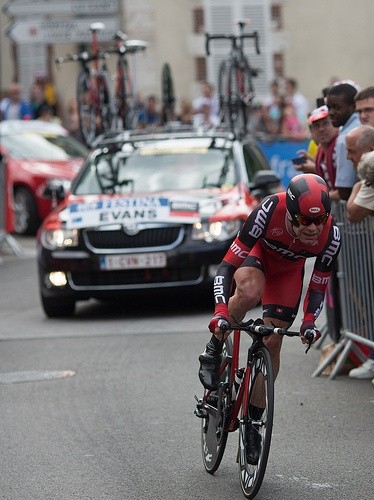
<box><xmin>194</xmin><ymin>318</ymin><xmax>315</xmax><ymax>500</ymax></box>
<box><xmin>161</xmin><ymin>61</ymin><xmax>176</xmax><ymax>126</ymax></box>
<box><xmin>53</xmin><ymin>20</ymin><xmax>148</xmax><ymax>151</ymax></box>
<box><xmin>203</xmin><ymin>18</ymin><xmax>261</xmax><ymax>141</ymax></box>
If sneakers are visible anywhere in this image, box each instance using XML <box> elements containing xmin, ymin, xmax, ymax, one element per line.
<box><xmin>245</xmin><ymin>428</ymin><xmax>260</xmax><ymax>464</ymax></box>
<box><xmin>197</xmin><ymin>345</ymin><xmax>221</xmax><ymax>391</ymax></box>
<box><xmin>349</xmin><ymin>358</ymin><xmax>374</xmax><ymax>380</ymax></box>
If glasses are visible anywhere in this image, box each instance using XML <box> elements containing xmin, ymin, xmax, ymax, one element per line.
<box><xmin>295</xmin><ymin>213</ymin><xmax>330</xmax><ymax>226</ymax></box>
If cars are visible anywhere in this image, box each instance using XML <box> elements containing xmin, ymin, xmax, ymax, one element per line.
<box><xmin>35</xmin><ymin>126</ymin><xmax>289</xmax><ymax>319</ymax></box>
<box><xmin>0</xmin><ymin>118</ymin><xmax>94</xmax><ymax>237</ymax></box>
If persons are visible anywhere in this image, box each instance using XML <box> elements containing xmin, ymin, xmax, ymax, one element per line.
<box><xmin>66</xmin><ymin>99</ymin><xmax>80</xmax><ymax>131</ymax></box>
<box><xmin>20</xmin><ymin>85</ymin><xmax>45</xmax><ymax>120</ymax></box>
<box><xmin>136</xmin><ymin>96</ymin><xmax>164</xmax><ymax>129</ymax></box>
<box><xmin>192</xmin><ymin>82</ymin><xmax>220</xmax><ymax>128</ymax></box>
<box><xmin>178</xmin><ymin>99</ymin><xmax>194</xmax><ymax>125</ymax></box>
<box><xmin>294</xmin><ymin>82</ymin><xmax>374</xmax><ymax>386</ymax></box>
<box><xmin>200</xmin><ymin>173</ymin><xmax>342</xmax><ymax>464</ymax></box>
<box><xmin>0</xmin><ymin>83</ymin><xmax>22</xmax><ymax>120</ymax></box>
<box><xmin>251</xmin><ymin>80</ymin><xmax>309</xmax><ymax>140</ymax></box>
<box><xmin>36</xmin><ymin>102</ymin><xmax>61</xmax><ymax>125</ymax></box>
<box><xmin>35</xmin><ymin>71</ymin><xmax>56</xmax><ymax>106</ymax></box>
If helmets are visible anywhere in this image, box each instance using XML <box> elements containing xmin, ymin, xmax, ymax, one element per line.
<box><xmin>285</xmin><ymin>174</ymin><xmax>329</xmax><ymax>217</ymax></box>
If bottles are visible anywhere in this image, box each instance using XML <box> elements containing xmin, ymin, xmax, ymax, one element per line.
<box><xmin>231</xmin><ymin>366</ymin><xmax>245</xmax><ymax>401</ymax></box>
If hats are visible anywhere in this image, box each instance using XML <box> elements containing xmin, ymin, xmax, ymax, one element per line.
<box><xmin>308</xmin><ymin>105</ymin><xmax>328</xmax><ymax>122</ymax></box>
